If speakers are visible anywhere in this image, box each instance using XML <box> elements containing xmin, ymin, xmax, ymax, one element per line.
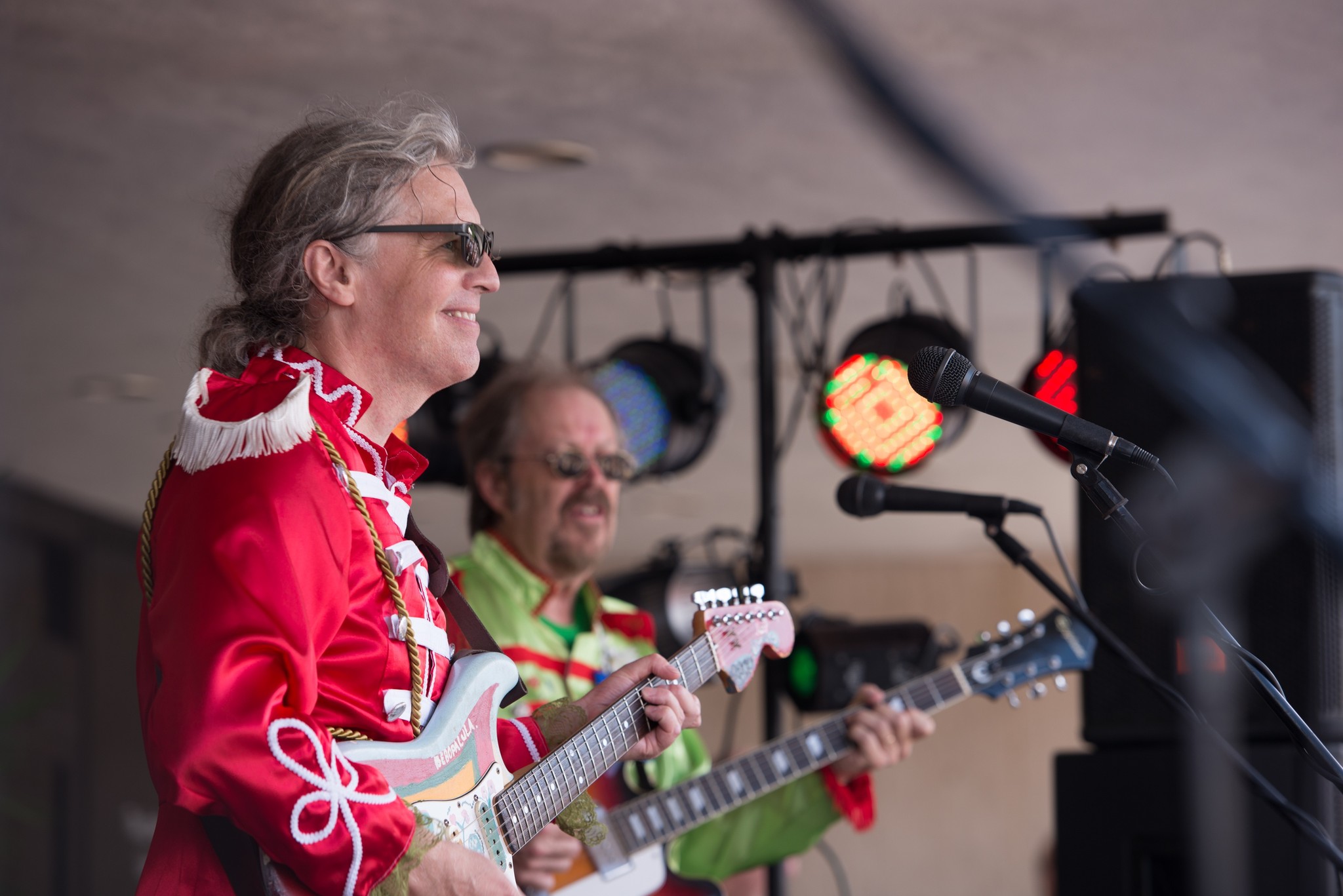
<box><xmin>1054</xmin><ymin>263</ymin><xmax>1343</xmax><ymax>896</ymax></box>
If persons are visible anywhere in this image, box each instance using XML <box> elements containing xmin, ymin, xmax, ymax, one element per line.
<box><xmin>435</xmin><ymin>363</ymin><xmax>940</xmax><ymax>895</ymax></box>
<box><xmin>127</xmin><ymin>87</ymin><xmax>705</xmax><ymax>896</ymax></box>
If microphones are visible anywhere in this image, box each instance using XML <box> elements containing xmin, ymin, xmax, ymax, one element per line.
<box><xmin>907</xmin><ymin>344</ymin><xmax>1160</xmax><ymax>472</ymax></box>
<box><xmin>835</xmin><ymin>471</ymin><xmax>1045</xmax><ymax>518</ymax></box>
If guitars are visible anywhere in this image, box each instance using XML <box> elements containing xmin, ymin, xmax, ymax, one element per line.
<box><xmin>204</xmin><ymin>583</ymin><xmax>795</xmax><ymax>896</ymax></box>
<box><xmin>510</xmin><ymin>607</ymin><xmax>1096</xmax><ymax>896</ymax></box>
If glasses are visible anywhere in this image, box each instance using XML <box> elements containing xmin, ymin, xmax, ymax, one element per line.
<box><xmin>505</xmin><ymin>451</ymin><xmax>635</xmax><ymax>479</ymax></box>
<box><xmin>361</xmin><ymin>222</ymin><xmax>495</xmax><ymax>269</ymax></box>
